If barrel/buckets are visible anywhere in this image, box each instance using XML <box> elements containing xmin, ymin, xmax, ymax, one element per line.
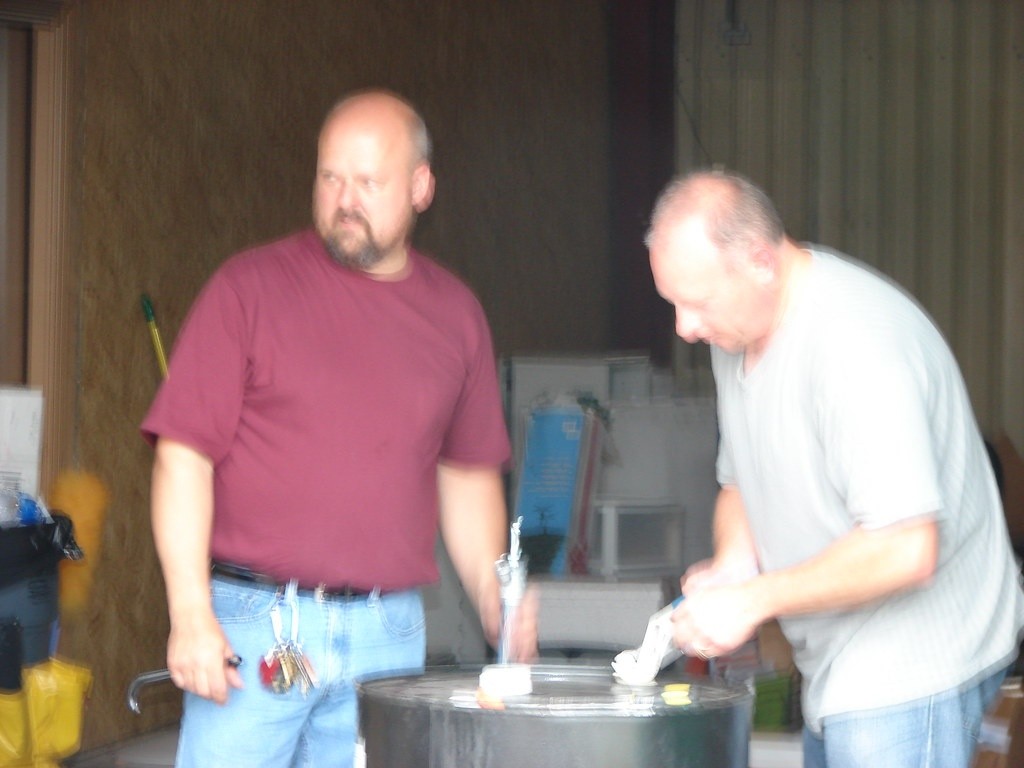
<box><xmin>361</xmin><ymin>665</ymin><xmax>750</xmax><ymax>768</ymax></box>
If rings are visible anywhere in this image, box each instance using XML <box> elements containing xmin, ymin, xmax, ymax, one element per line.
<box><xmin>225</xmin><ymin>654</ymin><xmax>242</xmax><ymax>668</ymax></box>
<box><xmin>693</xmin><ymin>637</ymin><xmax>711</xmax><ymax>652</ymax></box>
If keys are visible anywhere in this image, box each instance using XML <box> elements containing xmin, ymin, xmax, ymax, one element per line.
<box><xmin>263</xmin><ymin>642</ymin><xmax>318</xmax><ymax>699</ymax></box>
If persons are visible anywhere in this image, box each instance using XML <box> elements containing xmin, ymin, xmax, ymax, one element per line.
<box><xmin>140</xmin><ymin>92</ymin><xmax>539</xmax><ymax>768</ymax></box>
<box><xmin>644</xmin><ymin>171</ymin><xmax>1024</xmax><ymax>768</ymax></box>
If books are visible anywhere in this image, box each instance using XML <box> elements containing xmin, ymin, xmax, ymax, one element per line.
<box><xmin>513</xmin><ymin>407</ymin><xmax>598</xmax><ymax>579</ymax></box>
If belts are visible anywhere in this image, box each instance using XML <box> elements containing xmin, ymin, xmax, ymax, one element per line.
<box><xmin>211</xmin><ymin>562</ymin><xmax>381</xmax><ymax>598</ymax></box>
